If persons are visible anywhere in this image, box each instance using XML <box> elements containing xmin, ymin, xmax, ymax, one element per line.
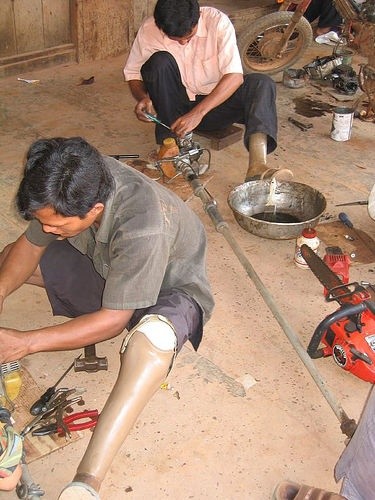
<box><xmin>123</xmin><ymin>0</ymin><xmax>293</xmax><ymax>182</ymax></box>
<box><xmin>0</xmin><ymin>136</ymin><xmax>215</xmax><ymax>499</ymax></box>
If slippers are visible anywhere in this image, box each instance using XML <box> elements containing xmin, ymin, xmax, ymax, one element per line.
<box><xmin>274</xmin><ymin>480</ymin><xmax>347</xmax><ymax>500</ymax></box>
<box><xmin>147</xmin><ymin>145</ymin><xmax>162</xmax><ymax>165</ymax></box>
<box><xmin>245</xmin><ymin>168</ymin><xmax>293</xmax><ymax>182</ymax></box>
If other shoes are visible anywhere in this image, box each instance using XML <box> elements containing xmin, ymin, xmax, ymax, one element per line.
<box><xmin>58</xmin><ymin>482</ymin><xmax>101</xmax><ymax>500</ymax></box>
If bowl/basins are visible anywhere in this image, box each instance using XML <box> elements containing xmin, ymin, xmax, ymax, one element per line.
<box><xmin>227</xmin><ymin>179</ymin><xmax>328</xmax><ymax>240</ymax></box>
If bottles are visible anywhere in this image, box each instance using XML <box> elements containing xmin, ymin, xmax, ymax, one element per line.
<box><xmin>293</xmin><ymin>224</ymin><xmax>321</xmax><ymax>270</ymax></box>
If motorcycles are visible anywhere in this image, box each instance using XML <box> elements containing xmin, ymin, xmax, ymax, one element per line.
<box><xmin>237</xmin><ymin>0</ymin><xmax>375</xmax><ymax>74</ymax></box>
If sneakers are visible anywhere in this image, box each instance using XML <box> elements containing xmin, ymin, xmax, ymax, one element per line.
<box><xmin>315</xmin><ymin>31</ymin><xmax>347</xmax><ymax>46</ymax></box>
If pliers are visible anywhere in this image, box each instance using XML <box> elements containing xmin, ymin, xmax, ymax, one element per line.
<box><xmin>20</xmin><ymin>389</ymin><xmax>99</xmax><ymax>442</ymax></box>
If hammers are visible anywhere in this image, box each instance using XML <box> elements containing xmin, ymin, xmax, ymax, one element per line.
<box><xmin>74</xmin><ymin>343</ymin><xmax>108</xmax><ymax>373</ymax></box>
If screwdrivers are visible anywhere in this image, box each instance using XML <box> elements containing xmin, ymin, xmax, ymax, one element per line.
<box><xmin>145</xmin><ymin>113</ymin><xmax>172</xmax><ymax>132</ymax></box>
<box><xmin>30</xmin><ymin>354</ymin><xmax>82</xmax><ymax>416</ymax></box>
<box><xmin>339</xmin><ymin>212</ymin><xmax>375</xmax><ymax>255</ymax></box>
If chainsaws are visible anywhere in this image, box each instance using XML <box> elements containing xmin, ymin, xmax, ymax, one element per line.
<box><xmin>300</xmin><ymin>243</ymin><xmax>375</xmax><ymax>384</ymax></box>
